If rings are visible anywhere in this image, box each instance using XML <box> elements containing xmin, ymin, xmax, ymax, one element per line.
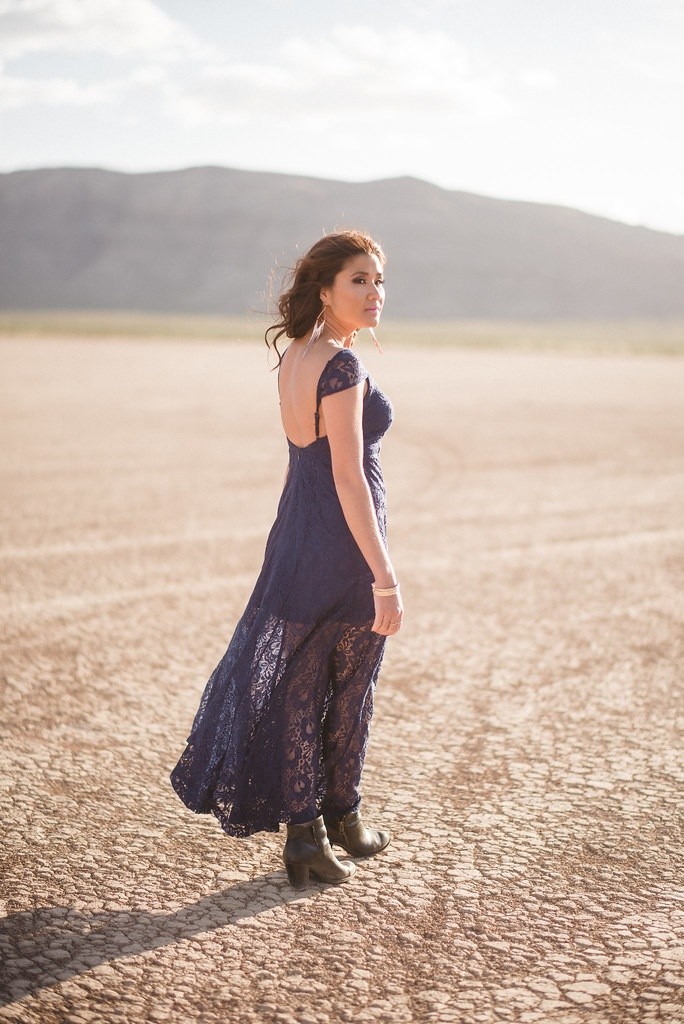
<box><xmin>391</xmin><ymin>622</ymin><xmax>402</xmax><ymax>626</ymax></box>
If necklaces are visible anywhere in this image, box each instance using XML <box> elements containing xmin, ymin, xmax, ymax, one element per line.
<box><xmin>324</xmin><ymin>328</ymin><xmax>341</xmax><ymax>347</ymax></box>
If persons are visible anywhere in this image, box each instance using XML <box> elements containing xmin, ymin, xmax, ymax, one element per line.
<box><xmin>170</xmin><ymin>233</ymin><xmax>403</xmax><ymax>890</ymax></box>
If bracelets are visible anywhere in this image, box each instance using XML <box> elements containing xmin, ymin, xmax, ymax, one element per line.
<box><xmin>373</xmin><ymin>583</ymin><xmax>400</xmax><ymax>596</ymax></box>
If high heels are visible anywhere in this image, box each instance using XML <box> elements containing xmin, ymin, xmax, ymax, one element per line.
<box><xmin>324</xmin><ymin>809</ymin><xmax>392</xmax><ymax>859</ymax></box>
<box><xmin>283</xmin><ymin>815</ymin><xmax>356</xmax><ymax>891</ymax></box>
